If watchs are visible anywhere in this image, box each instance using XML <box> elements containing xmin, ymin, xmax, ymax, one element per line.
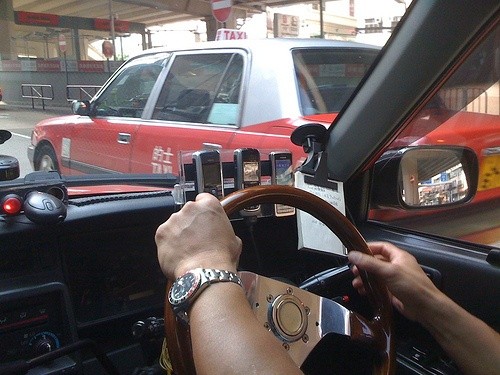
<box><xmin>167</xmin><ymin>267</ymin><xmax>245</xmax><ymax>326</ymax></box>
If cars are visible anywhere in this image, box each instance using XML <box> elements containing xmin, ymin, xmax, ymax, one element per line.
<box><xmin>26</xmin><ymin>28</ymin><xmax>499</xmax><ymax>239</ymax></box>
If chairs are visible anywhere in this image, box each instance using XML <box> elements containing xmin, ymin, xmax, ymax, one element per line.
<box><xmin>177</xmin><ymin>89</ymin><xmax>211</xmax><ymax>108</ymax></box>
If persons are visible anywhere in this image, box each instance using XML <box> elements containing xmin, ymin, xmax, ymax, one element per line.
<box><xmin>152</xmin><ymin>191</ymin><xmax>500</xmax><ymax>374</ymax></box>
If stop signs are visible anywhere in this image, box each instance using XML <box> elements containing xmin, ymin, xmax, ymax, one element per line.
<box><xmin>211</xmin><ymin>0</ymin><xmax>232</xmax><ymax>22</ymax></box>
<box><xmin>58</xmin><ymin>34</ymin><xmax>66</xmax><ymax>52</ymax></box>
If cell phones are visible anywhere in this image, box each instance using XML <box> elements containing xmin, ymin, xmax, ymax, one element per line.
<box><xmin>192</xmin><ymin>150</ymin><xmax>224</xmax><ymax>200</ymax></box>
<box><xmin>269</xmin><ymin>151</ymin><xmax>296</xmax><ymax>218</ymax></box>
<box><xmin>234</xmin><ymin>148</ymin><xmax>261</xmax><ymax>217</ymax></box>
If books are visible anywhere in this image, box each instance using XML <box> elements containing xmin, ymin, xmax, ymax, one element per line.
<box><xmin>293</xmin><ymin>170</ymin><xmax>348</xmax><ymax>258</ymax></box>
